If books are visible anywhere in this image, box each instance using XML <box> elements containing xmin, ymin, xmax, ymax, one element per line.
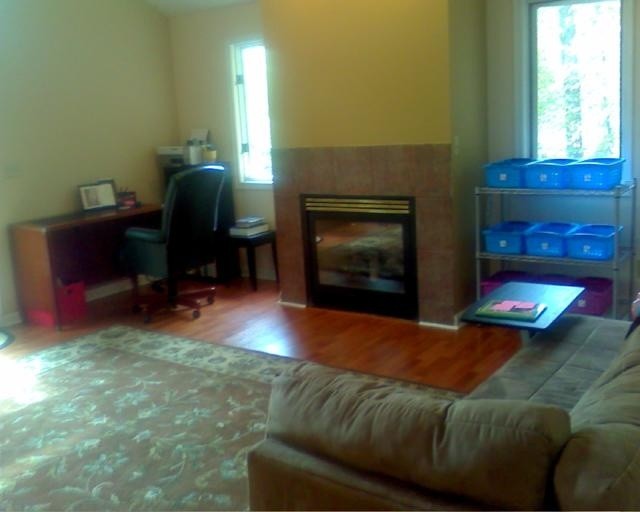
<box><xmin>473</xmin><ymin>299</ymin><xmax>547</xmax><ymax>322</ymax></box>
<box><xmin>229</xmin><ymin>215</ymin><xmax>270</xmax><ymax>237</ymax></box>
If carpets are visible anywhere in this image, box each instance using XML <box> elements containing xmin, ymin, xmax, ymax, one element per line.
<box><xmin>0</xmin><ymin>324</ymin><xmax>467</xmax><ymax>512</ymax></box>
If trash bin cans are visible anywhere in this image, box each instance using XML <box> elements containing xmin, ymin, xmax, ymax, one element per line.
<box><xmin>54</xmin><ymin>272</ymin><xmax>87</xmax><ymax>325</ymax></box>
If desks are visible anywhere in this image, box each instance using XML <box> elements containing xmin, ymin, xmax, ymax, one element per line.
<box><xmin>460</xmin><ymin>282</ymin><xmax>586</xmax><ymax>350</ymax></box>
<box><xmin>10</xmin><ymin>203</ymin><xmax>163</xmax><ymax>325</ymax></box>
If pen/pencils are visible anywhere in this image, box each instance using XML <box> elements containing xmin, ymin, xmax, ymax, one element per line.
<box><xmin>119</xmin><ymin>186</ymin><xmax>129</xmax><ymax>194</ymax></box>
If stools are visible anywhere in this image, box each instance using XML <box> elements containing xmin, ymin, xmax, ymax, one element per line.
<box><xmin>226</xmin><ymin>230</ymin><xmax>278</xmax><ymax>290</ymax></box>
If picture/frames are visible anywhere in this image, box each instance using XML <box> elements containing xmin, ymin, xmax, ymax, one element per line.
<box><xmin>77</xmin><ymin>178</ymin><xmax>120</xmax><ymax>212</ymax></box>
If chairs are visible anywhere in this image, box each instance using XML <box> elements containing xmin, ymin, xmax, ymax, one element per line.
<box><xmin>125</xmin><ymin>163</ymin><xmax>229</xmax><ymax>324</ymax></box>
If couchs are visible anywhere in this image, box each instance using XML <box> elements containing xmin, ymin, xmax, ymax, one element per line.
<box><xmin>247</xmin><ymin>312</ymin><xmax>639</xmax><ymax>510</ymax></box>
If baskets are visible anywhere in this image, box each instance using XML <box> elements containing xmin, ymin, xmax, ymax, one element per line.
<box><xmin>481</xmin><ymin>157</ymin><xmax>627</xmax><ymax>190</ymax></box>
<box><xmin>481</xmin><ymin>219</ymin><xmax>624</xmax><ymax>260</ymax></box>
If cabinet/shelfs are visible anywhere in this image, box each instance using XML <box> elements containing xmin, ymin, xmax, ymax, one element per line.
<box><xmin>475</xmin><ymin>180</ymin><xmax>636</xmax><ymax>319</ymax></box>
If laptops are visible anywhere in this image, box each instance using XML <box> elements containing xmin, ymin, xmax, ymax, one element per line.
<box><xmin>78</xmin><ymin>180</ymin><xmax>127</xmax><ymax>216</ymax></box>
